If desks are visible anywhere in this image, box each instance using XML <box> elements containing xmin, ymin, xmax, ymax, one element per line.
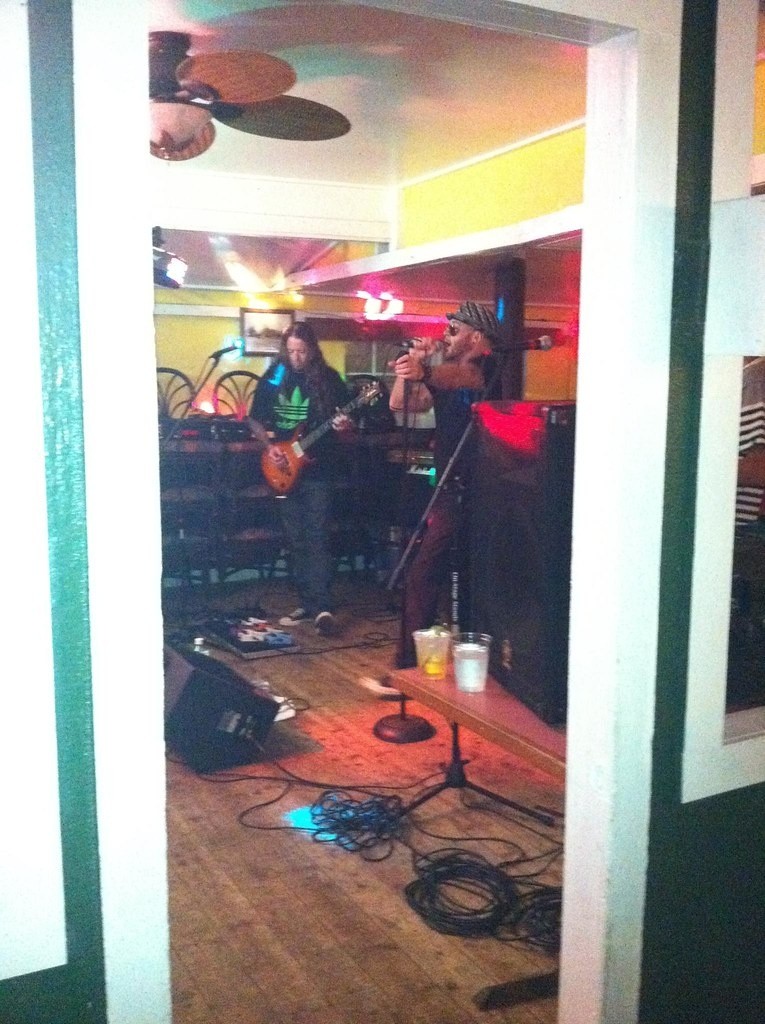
<box><xmin>369</xmin><ymin>658</ymin><xmax>566</xmax><ymax>1009</ymax></box>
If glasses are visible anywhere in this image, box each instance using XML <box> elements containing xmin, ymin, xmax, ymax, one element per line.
<box><xmin>445</xmin><ymin>324</ymin><xmax>479</xmax><ymax>336</ymax></box>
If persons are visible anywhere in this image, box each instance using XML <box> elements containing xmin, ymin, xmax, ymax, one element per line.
<box><xmin>247</xmin><ymin>320</ymin><xmax>356</xmax><ymax>635</ymax></box>
<box><xmin>359</xmin><ymin>300</ymin><xmax>503</xmax><ymax>695</ymax></box>
<box><xmin>726</xmin><ymin>355</ymin><xmax>765</xmax><ymax>712</ymax></box>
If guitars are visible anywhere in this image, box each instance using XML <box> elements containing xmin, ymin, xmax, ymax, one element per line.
<box><xmin>259</xmin><ymin>379</ymin><xmax>385</xmax><ymax>494</ymax></box>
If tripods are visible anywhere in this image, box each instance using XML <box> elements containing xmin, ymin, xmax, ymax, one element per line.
<box><xmin>373</xmin><ymin>351</ymin><xmax>556</xmax><ymax>838</ymax></box>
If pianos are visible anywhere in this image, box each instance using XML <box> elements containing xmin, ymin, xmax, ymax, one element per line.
<box><xmin>382</xmin><ymin>447</ymin><xmax>437</xmax><ymax>479</ymax></box>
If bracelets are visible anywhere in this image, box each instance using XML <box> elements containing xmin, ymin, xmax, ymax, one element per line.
<box><xmin>418</xmin><ymin>362</ymin><xmax>431</xmax><ymax>381</ymax></box>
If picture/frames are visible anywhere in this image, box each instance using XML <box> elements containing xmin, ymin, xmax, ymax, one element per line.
<box><xmin>240</xmin><ymin>307</ymin><xmax>295</xmax><ymax>357</ymax></box>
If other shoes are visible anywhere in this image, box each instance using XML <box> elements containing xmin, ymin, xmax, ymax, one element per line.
<box><xmin>360</xmin><ymin>675</ymin><xmax>401</xmax><ymax>696</ymax></box>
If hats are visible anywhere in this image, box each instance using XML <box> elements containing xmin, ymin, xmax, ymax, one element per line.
<box><xmin>446</xmin><ymin>301</ymin><xmax>504</xmax><ymax>347</ymax></box>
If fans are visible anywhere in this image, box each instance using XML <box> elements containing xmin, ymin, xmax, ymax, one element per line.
<box><xmin>148</xmin><ymin>31</ymin><xmax>352</xmax><ymax>161</ymax></box>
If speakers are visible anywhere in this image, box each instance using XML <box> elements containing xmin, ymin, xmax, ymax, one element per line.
<box><xmin>160</xmin><ymin>642</ymin><xmax>281</xmax><ymax>763</ymax></box>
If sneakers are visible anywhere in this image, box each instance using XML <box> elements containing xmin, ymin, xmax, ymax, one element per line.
<box><xmin>280</xmin><ymin>606</ymin><xmax>314</xmax><ymax>626</ymax></box>
<box><xmin>313</xmin><ymin>610</ymin><xmax>334</xmax><ymax>637</ymax></box>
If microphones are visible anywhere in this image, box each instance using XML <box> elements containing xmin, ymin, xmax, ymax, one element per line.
<box><xmin>490</xmin><ymin>336</ymin><xmax>553</xmax><ymax>352</ymax></box>
<box><xmin>396</xmin><ymin>339</ymin><xmax>444</xmax><ymax>352</ymax></box>
<box><xmin>209</xmin><ymin>339</ymin><xmax>245</xmax><ymax>358</ymax></box>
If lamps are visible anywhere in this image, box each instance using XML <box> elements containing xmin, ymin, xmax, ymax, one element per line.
<box><xmin>153</xmin><ymin>254</ymin><xmax>188</xmax><ymax>289</ymax></box>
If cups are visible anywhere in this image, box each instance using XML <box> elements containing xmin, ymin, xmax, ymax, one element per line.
<box><xmin>449</xmin><ymin>632</ymin><xmax>495</xmax><ymax>693</ymax></box>
<box><xmin>411</xmin><ymin>629</ymin><xmax>452</xmax><ymax>680</ymax></box>
